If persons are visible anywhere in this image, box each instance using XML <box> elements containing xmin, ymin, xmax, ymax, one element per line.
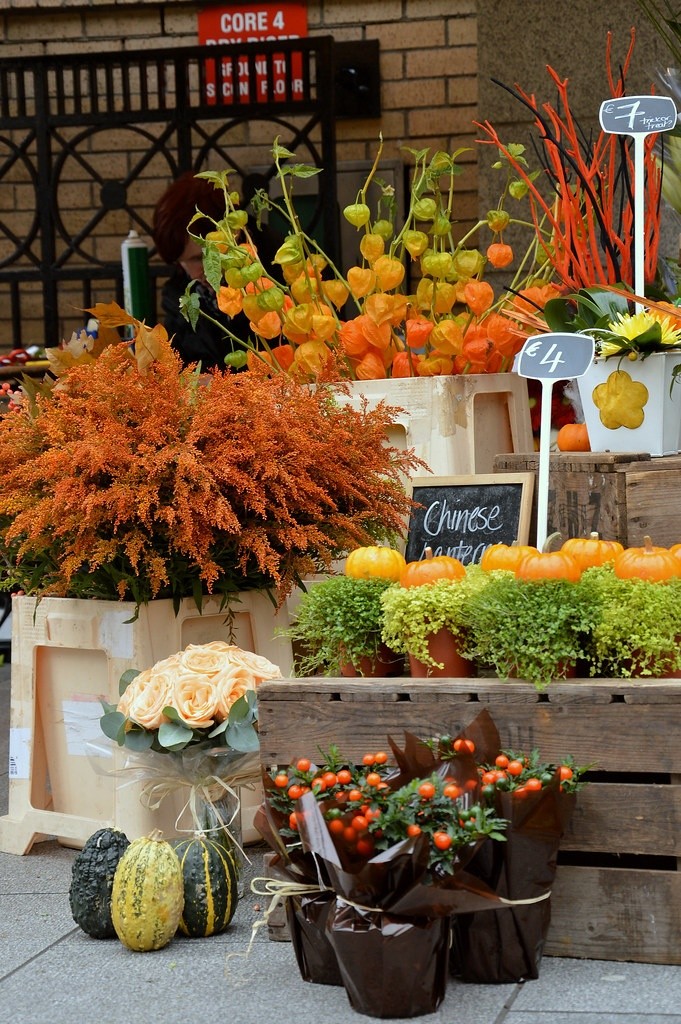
<box><xmin>150</xmin><ymin>173</ymin><xmax>291</xmax><ymax>373</ymax></box>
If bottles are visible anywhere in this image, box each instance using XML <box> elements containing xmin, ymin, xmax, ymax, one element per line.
<box><xmin>121</xmin><ymin>230</ymin><xmax>153</xmax><ymax>339</ymax></box>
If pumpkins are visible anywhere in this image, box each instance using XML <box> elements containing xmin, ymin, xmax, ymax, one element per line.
<box><xmin>346</xmin><ymin>545</ymin><xmax>406</xmax><ymax>583</ymax></box>
<box><xmin>557</xmin><ymin>421</ymin><xmax>590</xmax><ymax>452</ymax></box>
<box><xmin>67</xmin><ymin>825</ymin><xmax>239</xmax><ymax>952</ymax></box>
<box><xmin>480</xmin><ymin>530</ymin><xmax>681</xmax><ymax>586</ymax></box>
<box><xmin>399</xmin><ymin>546</ymin><xmax>467</xmax><ymax>589</ymax></box>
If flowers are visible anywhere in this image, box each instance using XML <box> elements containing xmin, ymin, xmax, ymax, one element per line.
<box><xmin>544</xmin><ymin>284</ymin><xmax>680</xmax><ymax>379</ymax></box>
<box><xmin>95</xmin><ymin>641</ymin><xmax>284</xmax><ymax>788</ymax></box>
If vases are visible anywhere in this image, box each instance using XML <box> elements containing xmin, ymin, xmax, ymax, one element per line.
<box><xmin>187</xmin><ymin>784</ymin><xmax>244</xmax><ymax>901</ymax></box>
<box><xmin>576</xmin><ymin>351</ymin><xmax>680</xmax><ymax>457</ymax></box>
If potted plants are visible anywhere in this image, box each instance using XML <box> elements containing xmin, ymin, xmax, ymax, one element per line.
<box><xmin>176</xmin><ymin>132</ymin><xmax>608</xmax><ymax>576</ymax></box>
<box><xmin>271</xmin><ymin>574</ymin><xmax>681</xmax><ymax>690</ymax></box>
<box><xmin>0</xmin><ymin>299</ymin><xmax>436</xmax><ymax>859</ymax></box>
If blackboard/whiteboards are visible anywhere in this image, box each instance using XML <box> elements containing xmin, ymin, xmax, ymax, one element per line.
<box><xmin>397</xmin><ymin>471</ymin><xmax>535</xmax><ymax>565</ymax></box>
